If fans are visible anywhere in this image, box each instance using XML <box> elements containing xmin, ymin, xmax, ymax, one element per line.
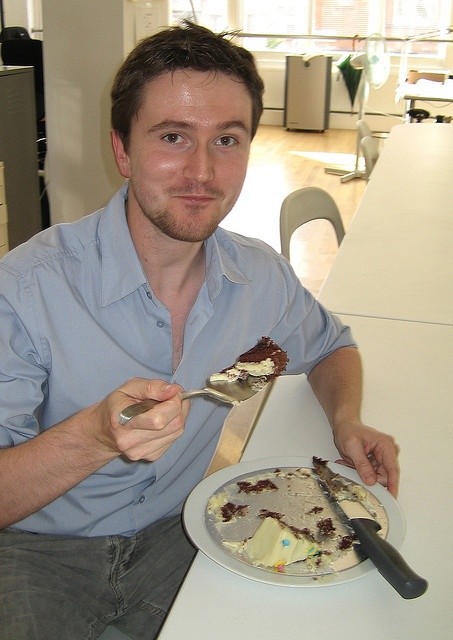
<box><xmin>324</xmin><ymin>32</ymin><xmax>390</xmax><ymax>183</ymax></box>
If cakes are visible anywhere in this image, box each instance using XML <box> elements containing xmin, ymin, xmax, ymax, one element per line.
<box><xmin>209</xmin><ymin>335</ymin><xmax>290</xmax><ymax>387</ymax></box>
<box><xmin>204</xmin><ymin>466</ymin><xmax>385</xmax><ymax>578</ymax></box>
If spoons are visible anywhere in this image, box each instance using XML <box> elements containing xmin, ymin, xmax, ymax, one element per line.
<box><xmin>118</xmin><ymin>364</ymin><xmax>271</xmax><ymax>427</ymax></box>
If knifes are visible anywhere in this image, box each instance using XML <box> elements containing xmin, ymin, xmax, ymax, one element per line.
<box><xmin>312</xmin><ymin>456</ymin><xmax>429</xmax><ymax>599</ymax></box>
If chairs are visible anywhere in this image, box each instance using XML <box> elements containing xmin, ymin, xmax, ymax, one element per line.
<box><xmin>279</xmin><ymin>187</ymin><xmax>346</xmax><ymax>263</ymax></box>
<box><xmin>357</xmin><ymin>120</ymin><xmax>373</xmax><ymax>139</ymax></box>
<box><xmin>360</xmin><ymin>136</ymin><xmax>379</xmax><ymax>176</ymax></box>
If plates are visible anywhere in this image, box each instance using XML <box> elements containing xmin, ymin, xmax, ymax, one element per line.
<box><xmin>182</xmin><ymin>456</ymin><xmax>407</xmax><ymax>587</ymax></box>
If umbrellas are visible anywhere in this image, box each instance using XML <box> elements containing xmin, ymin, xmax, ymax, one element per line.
<box><xmin>335</xmin><ymin>34</ymin><xmax>362</xmax><ymax>116</ymax></box>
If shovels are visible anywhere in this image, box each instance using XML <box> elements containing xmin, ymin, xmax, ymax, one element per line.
<box><xmin>117</xmin><ymin>338</ymin><xmax>288</xmax><ymax>425</ymax></box>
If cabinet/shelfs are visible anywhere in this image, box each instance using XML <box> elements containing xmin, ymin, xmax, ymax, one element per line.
<box><xmin>1</xmin><ymin>65</ymin><xmax>42</xmax><ymax>250</ymax></box>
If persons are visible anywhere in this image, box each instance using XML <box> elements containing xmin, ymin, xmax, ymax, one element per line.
<box><xmin>0</xmin><ymin>14</ymin><xmax>401</xmax><ymax>640</ymax></box>
